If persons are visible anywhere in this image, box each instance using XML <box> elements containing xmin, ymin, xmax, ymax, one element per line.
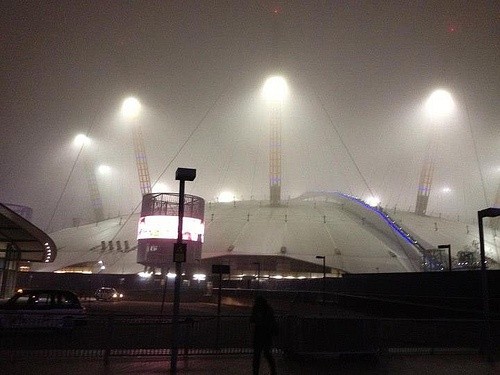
<box><xmin>249</xmin><ymin>295</ymin><xmax>280</xmax><ymax>375</ymax></box>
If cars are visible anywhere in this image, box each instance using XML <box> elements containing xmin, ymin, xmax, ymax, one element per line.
<box><xmin>3</xmin><ymin>287</ymin><xmax>89</xmax><ymax>329</ymax></box>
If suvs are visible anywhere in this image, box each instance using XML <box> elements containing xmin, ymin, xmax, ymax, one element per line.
<box><xmin>94</xmin><ymin>286</ymin><xmax>123</xmax><ymax>299</ymax></box>
